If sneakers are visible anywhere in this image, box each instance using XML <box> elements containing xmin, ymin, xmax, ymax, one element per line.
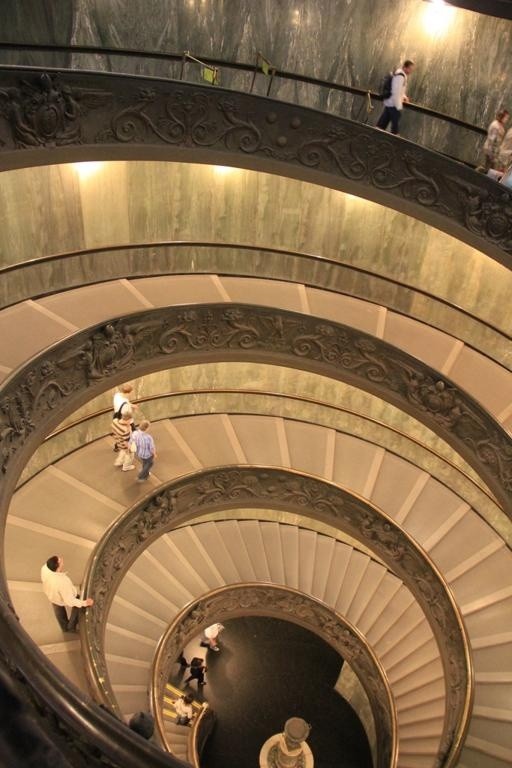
<box><xmin>122</xmin><ymin>465</ymin><xmax>135</xmax><ymax>472</ymax></box>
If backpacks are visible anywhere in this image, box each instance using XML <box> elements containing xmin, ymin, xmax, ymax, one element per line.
<box><xmin>378</xmin><ymin>68</ymin><xmax>405</xmax><ymax>99</ymax></box>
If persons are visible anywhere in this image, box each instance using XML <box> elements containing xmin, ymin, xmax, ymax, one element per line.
<box><xmin>113</xmin><ymin>384</ymin><xmax>138</xmax><ymax>452</ymax></box>
<box><xmin>124</xmin><ymin>421</ymin><xmax>159</xmax><ymax>484</ymax></box>
<box><xmin>376</xmin><ymin>59</ymin><xmax>416</xmax><ymax>136</ymax></box>
<box><xmin>203</xmin><ymin>623</ymin><xmax>226</xmax><ymax>652</ymax></box>
<box><xmin>494</xmin><ymin>127</ymin><xmax>511</xmax><ymax>175</ymax></box>
<box><xmin>175</xmin><ymin>651</ymin><xmax>190</xmax><ymax>667</ymax></box>
<box><xmin>173</xmin><ymin>692</ymin><xmax>194</xmax><ymax>726</ymax></box>
<box><xmin>473</xmin><ymin>108</ymin><xmax>510</xmax><ymax>175</ymax></box>
<box><xmin>40</xmin><ymin>555</ymin><xmax>96</xmax><ymax>633</ymax></box>
<box><xmin>183</xmin><ymin>657</ymin><xmax>209</xmax><ymax>685</ymax></box>
<box><xmin>111</xmin><ymin>404</ymin><xmax>137</xmax><ymax>472</ymax></box>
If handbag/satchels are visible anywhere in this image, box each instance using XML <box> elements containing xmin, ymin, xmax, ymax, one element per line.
<box><xmin>113</xmin><ymin>402</ymin><xmax>128</xmax><ymax>419</ymax></box>
